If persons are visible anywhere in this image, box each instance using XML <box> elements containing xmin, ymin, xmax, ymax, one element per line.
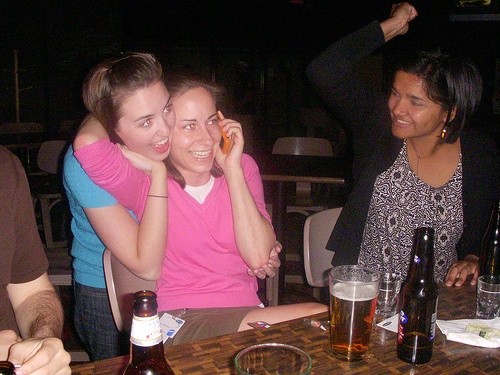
<box><xmin>73</xmin><ymin>74</ymin><xmax>329</xmax><ymax>346</ymax></box>
<box><xmin>319</xmin><ymin>1</ymin><xmax>500</xmax><ymax>289</ymax></box>
<box><xmin>0</xmin><ymin>141</ymin><xmax>73</xmax><ymax>375</ymax></box>
<box><xmin>61</xmin><ymin>52</ymin><xmax>282</xmax><ymax>359</ymax></box>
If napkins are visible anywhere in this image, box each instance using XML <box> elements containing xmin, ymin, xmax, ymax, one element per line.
<box><xmin>436</xmin><ymin>317</ymin><xmax>500</xmax><ymax>348</ymax></box>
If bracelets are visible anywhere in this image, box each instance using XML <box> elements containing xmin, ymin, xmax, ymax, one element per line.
<box><xmin>147</xmin><ymin>194</ymin><xmax>168</xmax><ymax>199</ymax></box>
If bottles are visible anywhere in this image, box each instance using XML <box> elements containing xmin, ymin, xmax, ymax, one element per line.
<box><xmin>122</xmin><ymin>290</ymin><xmax>175</xmax><ymax>375</ymax></box>
<box><xmin>396</xmin><ymin>227</ymin><xmax>440</xmax><ymax>364</ymax></box>
<box><xmin>480</xmin><ymin>200</ymin><xmax>500</xmax><ymax>286</ymax></box>
<box><xmin>0</xmin><ymin>361</ymin><xmax>14</xmax><ymax>375</ymax></box>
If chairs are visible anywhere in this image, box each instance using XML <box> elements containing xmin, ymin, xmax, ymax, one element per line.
<box><xmin>272</xmin><ymin>138</ymin><xmax>333</xmax><ymax>217</ymax></box>
<box><xmin>303</xmin><ymin>207</ymin><xmax>343</xmax><ymax>288</ymax></box>
<box><xmin>102</xmin><ymin>247</ymin><xmax>157</xmax><ymax>331</ymax></box>
<box><xmin>38</xmin><ymin>141</ymin><xmax>72</xmax><ymax>249</ymax></box>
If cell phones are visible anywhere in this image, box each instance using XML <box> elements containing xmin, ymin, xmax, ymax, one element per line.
<box><xmin>216</xmin><ymin>114</ymin><xmax>231</xmax><ymax>155</ymax></box>
<box><xmin>0</xmin><ymin>361</ymin><xmax>16</xmax><ymax>375</ymax></box>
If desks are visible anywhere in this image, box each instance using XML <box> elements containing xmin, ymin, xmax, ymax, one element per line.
<box><xmin>71</xmin><ymin>278</ymin><xmax>500</xmax><ymax>375</ymax></box>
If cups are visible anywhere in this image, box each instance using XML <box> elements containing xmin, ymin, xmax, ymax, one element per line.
<box><xmin>234</xmin><ymin>342</ymin><xmax>312</xmax><ymax>375</ymax></box>
<box><xmin>375</xmin><ymin>272</ymin><xmax>403</xmax><ymax>316</ymax></box>
<box><xmin>476</xmin><ymin>275</ymin><xmax>500</xmax><ymax>320</ymax></box>
<box><xmin>328</xmin><ymin>264</ymin><xmax>383</xmax><ymax>362</ymax></box>
